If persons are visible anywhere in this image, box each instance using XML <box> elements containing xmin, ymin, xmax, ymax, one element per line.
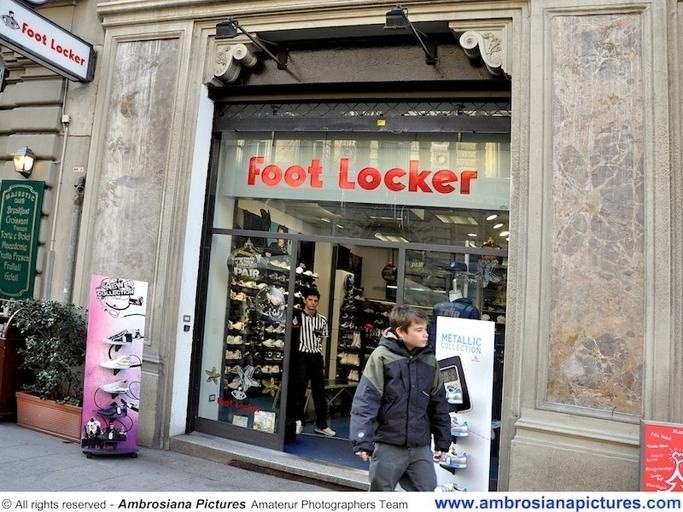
<box><xmin>428</xmin><ymin>289</ymin><xmax>480</xmax><ymax>351</ymax></box>
<box><xmin>347</xmin><ymin>303</ymin><xmax>452</xmax><ymax>491</ymax></box>
<box><xmin>268</xmin><ymin>224</ymin><xmax>291</xmax><ymax>256</ymax></box>
<box><xmin>289</xmin><ymin>287</ymin><xmax>337</xmax><ymax>437</ymax></box>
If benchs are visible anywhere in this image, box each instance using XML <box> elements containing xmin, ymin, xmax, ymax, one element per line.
<box><xmin>305</xmin><ymin>379</ymin><xmax>358</xmax><ymax>426</ymax></box>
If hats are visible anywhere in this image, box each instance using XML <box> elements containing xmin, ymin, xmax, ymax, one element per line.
<box><xmin>439</xmin><ymin>261</ymin><xmax>467</xmax><ymax>272</ymax></box>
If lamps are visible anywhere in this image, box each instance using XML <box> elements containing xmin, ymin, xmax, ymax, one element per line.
<box><xmin>9</xmin><ymin>145</ymin><xmax>37</xmax><ymax>178</ymax></box>
<box><xmin>215</xmin><ymin>16</ymin><xmax>289</xmax><ymax>69</ymax></box>
<box><xmin>384</xmin><ymin>3</ymin><xmax>439</xmax><ymax>65</ymax></box>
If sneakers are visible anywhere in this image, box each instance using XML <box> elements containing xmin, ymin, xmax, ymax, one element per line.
<box><xmin>225</xmin><ymin>260</ymin><xmax>320</xmax><ymax>389</ymax></box>
<box><xmin>445</xmin><ymin>384</ymin><xmax>462</xmax><ymax>404</ymax></box>
<box><xmin>100</xmin><ymin>381</ymin><xmax>128</xmax><ymax>394</ymax></box>
<box><xmin>450</xmin><ymin>417</ymin><xmax>469</xmax><ymax>437</ymax></box>
<box><xmin>97</xmin><ymin>404</ymin><xmax>128</xmax><ymax>416</ymax></box>
<box><xmin>439</xmin><ymin>449</ymin><xmax>467</xmax><ymax>469</ymax></box>
<box><xmin>434</xmin><ymin>484</ymin><xmax>467</xmax><ymax>492</ymax></box>
<box><xmin>340</xmin><ymin>306</ymin><xmax>389</xmax><ymax>347</ymax></box>
<box><xmin>105</xmin><ymin>330</ymin><xmax>132</xmax><ymax>345</ymax></box>
<box><xmin>295</xmin><ymin>421</ymin><xmax>303</xmax><ymax>434</ymax></box>
<box><xmin>100</xmin><ymin>357</ymin><xmax>129</xmax><ymax>369</ymax></box>
<box><xmin>97</xmin><ymin>427</ymin><xmax>126</xmax><ymax>441</ymax></box>
<box><xmin>314</xmin><ymin>426</ymin><xmax>335</xmax><ymax>436</ymax></box>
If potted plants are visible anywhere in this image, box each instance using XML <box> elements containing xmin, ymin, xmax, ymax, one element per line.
<box><xmin>5</xmin><ymin>297</ymin><xmax>89</xmax><ymax>444</ymax></box>
